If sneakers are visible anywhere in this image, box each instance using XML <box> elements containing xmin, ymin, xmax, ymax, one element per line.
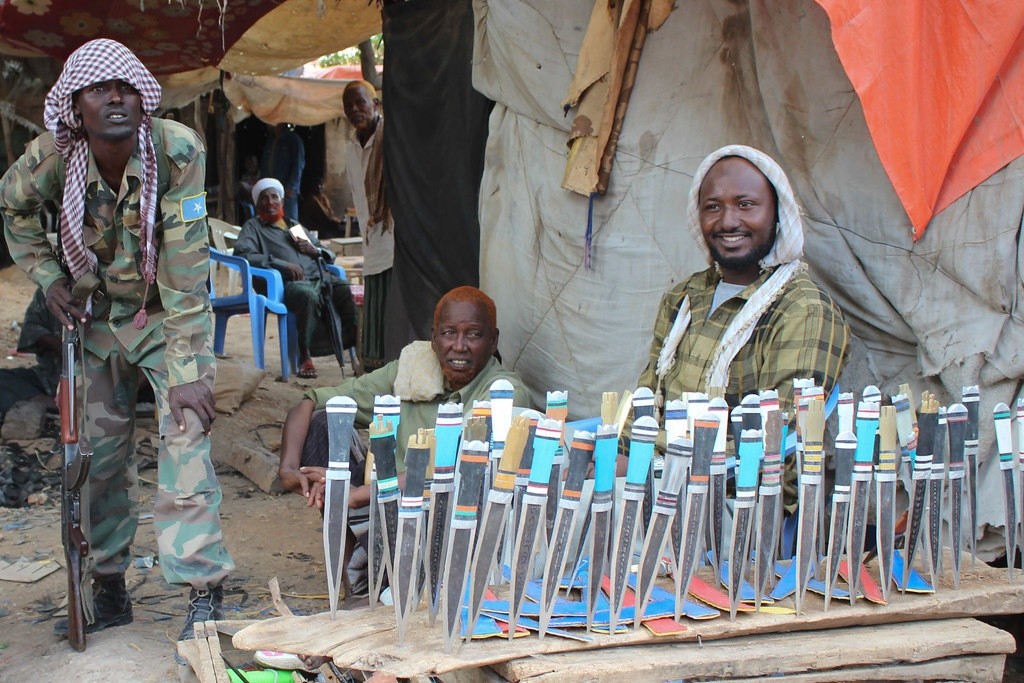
<box><xmin>175</xmin><ymin>585</ymin><xmax>224</xmax><ymax>668</ymax></box>
<box><xmin>55</xmin><ymin>575</ymin><xmax>133</xmax><ymax>637</ymax></box>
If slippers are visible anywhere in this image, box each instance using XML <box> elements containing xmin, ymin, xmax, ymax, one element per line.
<box><xmin>297</xmin><ymin>358</ymin><xmax>319</xmax><ymax>379</ymax></box>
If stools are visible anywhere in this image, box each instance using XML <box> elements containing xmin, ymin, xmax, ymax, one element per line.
<box><xmin>329</xmin><ymin>237</ymin><xmax>365</xmax><ymax>282</ymax></box>
<box><xmin>344</xmin><ymin>207</ymin><xmax>359</xmax><ymax>238</ymax></box>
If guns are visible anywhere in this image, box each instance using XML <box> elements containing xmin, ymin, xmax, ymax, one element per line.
<box><xmin>59</xmin><ymin>287</ymin><xmax>90</xmax><ymax>651</ymax></box>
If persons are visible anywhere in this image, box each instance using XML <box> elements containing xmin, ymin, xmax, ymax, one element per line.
<box><xmin>0</xmin><ymin>38</ymin><xmax>236</xmax><ymax>665</ymax></box>
<box><xmin>278</xmin><ymin>286</ymin><xmax>534</xmax><ymax>601</ymax></box>
<box><xmin>342</xmin><ymin>80</ymin><xmax>396</xmax><ymax>369</ymax></box>
<box><xmin>561</xmin><ymin>144</ymin><xmax>853</xmax><ymax>561</ymax></box>
<box><xmin>17</xmin><ymin>287</ymin><xmax>63</xmax><ymax>414</ymax></box>
<box><xmin>233</xmin><ymin>178</ymin><xmax>358</xmax><ymax>379</ymax></box>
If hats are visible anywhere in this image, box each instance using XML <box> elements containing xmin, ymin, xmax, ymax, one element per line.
<box><xmin>251</xmin><ymin>178</ymin><xmax>284</xmax><ymax>206</ymax></box>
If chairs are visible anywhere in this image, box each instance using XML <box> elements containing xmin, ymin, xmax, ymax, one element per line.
<box><xmin>288</xmin><ymin>263</ymin><xmax>355</xmax><ymax>374</ymax></box>
<box><xmin>208</xmin><ymin>247</ymin><xmax>288</xmax><ymax>380</ymax></box>
